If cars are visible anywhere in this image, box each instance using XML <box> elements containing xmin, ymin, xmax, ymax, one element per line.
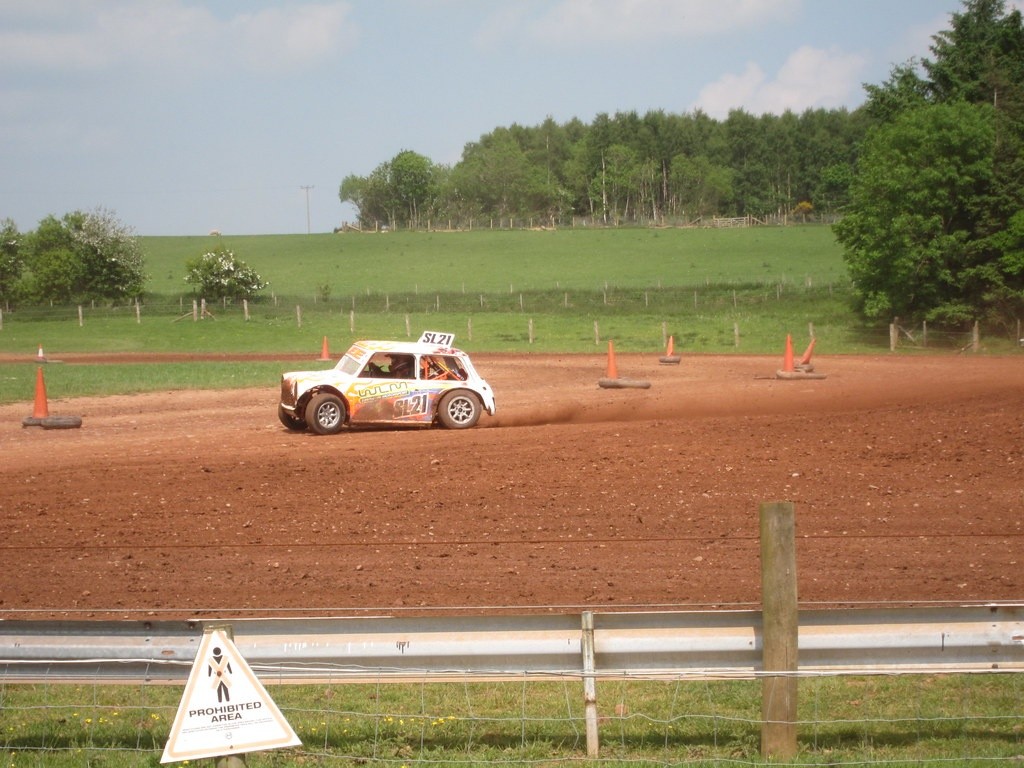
<box><xmin>278</xmin><ymin>342</ymin><xmax>500</xmax><ymax>434</ymax></box>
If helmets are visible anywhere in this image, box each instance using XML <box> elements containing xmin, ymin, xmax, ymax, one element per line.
<box><xmin>385</xmin><ymin>354</ymin><xmax>414</xmax><ymax>373</ymax></box>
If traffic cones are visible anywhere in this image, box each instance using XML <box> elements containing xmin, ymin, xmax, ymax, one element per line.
<box><xmin>316</xmin><ymin>335</ymin><xmax>333</xmax><ymax>362</ymax></box>
<box><xmin>782</xmin><ymin>333</ymin><xmax>801</xmax><ymax>374</ymax></box>
<box><xmin>665</xmin><ymin>335</ymin><xmax>675</xmax><ymax>358</ymax></box>
<box><xmin>605</xmin><ymin>341</ymin><xmax>620</xmax><ymax>381</ymax></box>
<box><xmin>797</xmin><ymin>339</ymin><xmax>817</xmax><ymax>364</ymax></box>
<box><xmin>24</xmin><ymin>371</ymin><xmax>55</xmax><ymax>429</ymax></box>
<box><xmin>35</xmin><ymin>343</ymin><xmax>48</xmax><ymax>365</ymax></box>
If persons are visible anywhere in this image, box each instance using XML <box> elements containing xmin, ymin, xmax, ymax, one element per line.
<box><xmin>369</xmin><ymin>352</ymin><xmax>409</xmax><ymax>378</ymax></box>
